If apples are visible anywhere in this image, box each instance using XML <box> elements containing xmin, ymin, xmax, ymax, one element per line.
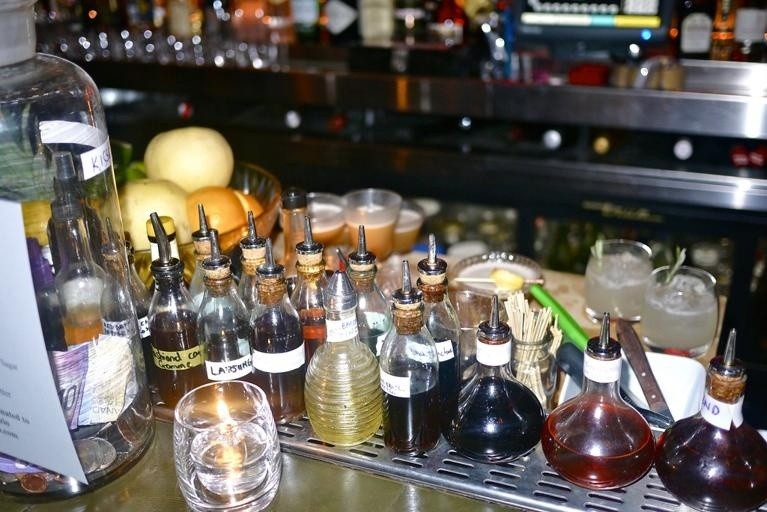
<box><xmin>117</xmin><ymin>127</ymin><xmax>234</xmax><ymax>252</ymax></box>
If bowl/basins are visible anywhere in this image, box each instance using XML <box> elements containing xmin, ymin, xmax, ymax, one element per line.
<box><xmin>283</xmin><ymin>191</ymin><xmax>348</xmax><ymax>244</ymax></box>
<box><xmin>113</xmin><ymin>164</ymin><xmax>283</xmax><ymax>280</ymax></box>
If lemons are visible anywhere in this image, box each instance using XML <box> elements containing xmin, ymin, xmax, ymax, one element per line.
<box><xmin>23</xmin><ymin>198</ymin><xmax>56</xmax><ymax>248</ymax></box>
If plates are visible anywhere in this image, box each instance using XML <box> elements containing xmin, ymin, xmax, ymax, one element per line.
<box><xmin>451</xmin><ymin>252</ymin><xmax>545</xmax><ymax>301</ymax></box>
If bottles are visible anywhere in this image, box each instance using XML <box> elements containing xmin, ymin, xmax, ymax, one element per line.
<box><xmin>98</xmin><ymin>203</ymin><xmax>764</xmax><ymax>512</ymax></box>
<box><xmin>0</xmin><ymin>0</ymin><xmax>157</xmax><ymax>500</ymax></box>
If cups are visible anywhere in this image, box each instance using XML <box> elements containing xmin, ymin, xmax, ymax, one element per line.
<box><xmin>640</xmin><ymin>267</ymin><xmax>720</xmax><ymax>362</ymax></box>
<box><xmin>170</xmin><ymin>379</ymin><xmax>280</xmax><ymax>512</ymax></box>
<box><xmin>343</xmin><ymin>189</ymin><xmax>426</xmax><ymax>260</ymax></box>
<box><xmin>580</xmin><ymin>238</ymin><xmax>653</xmax><ymax>326</ymax></box>
